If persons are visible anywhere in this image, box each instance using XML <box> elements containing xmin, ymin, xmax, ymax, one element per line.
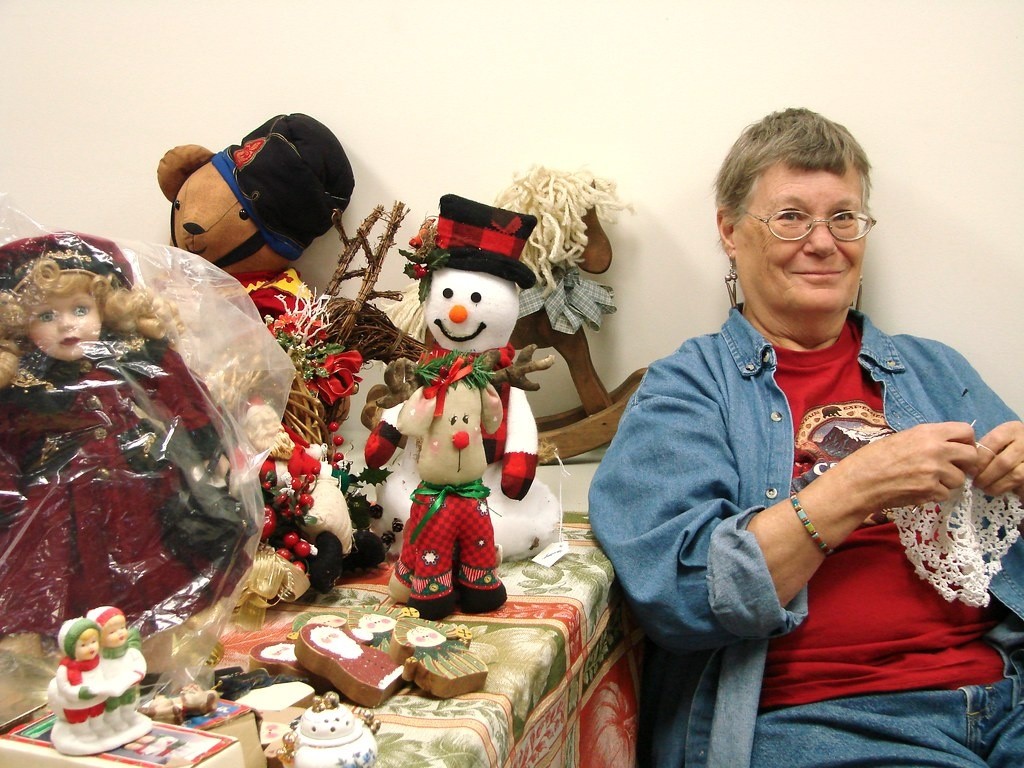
<box><xmin>247</xmin><ymin>405</ymin><xmax>321</xmax><ymax>509</ymax></box>
<box><xmin>0</xmin><ymin>231</ymin><xmax>255</xmax><ymax>657</ymax></box>
<box><xmin>588</xmin><ymin>114</ymin><xmax>1024</xmax><ymax>768</ymax></box>
<box><xmin>46</xmin><ymin>607</ymin><xmax>149</xmax><ymax>743</ymax></box>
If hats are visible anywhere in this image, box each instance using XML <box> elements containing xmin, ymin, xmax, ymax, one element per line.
<box><xmin>742</xmin><ymin>210</ymin><xmax>877</xmax><ymax>242</ymax></box>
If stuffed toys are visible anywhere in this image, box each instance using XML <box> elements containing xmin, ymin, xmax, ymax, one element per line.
<box><xmin>158</xmin><ymin>111</ymin><xmax>649</xmax><ymax>619</ymax></box>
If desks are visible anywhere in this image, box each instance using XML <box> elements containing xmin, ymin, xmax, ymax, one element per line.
<box><xmin>0</xmin><ymin>510</ymin><xmax>643</xmax><ymax>768</ymax></box>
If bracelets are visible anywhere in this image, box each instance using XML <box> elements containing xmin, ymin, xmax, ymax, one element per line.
<box><xmin>785</xmin><ymin>490</ymin><xmax>835</xmax><ymax>560</ymax></box>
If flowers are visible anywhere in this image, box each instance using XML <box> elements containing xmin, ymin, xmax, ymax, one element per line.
<box><xmin>263</xmin><ymin>280</ymin><xmax>365</xmax><ymax>406</ymax></box>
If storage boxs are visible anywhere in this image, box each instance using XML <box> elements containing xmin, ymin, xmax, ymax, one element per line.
<box><xmin>180</xmin><ymin>698</ymin><xmax>267</xmax><ymax>768</ymax></box>
<box><xmin>0</xmin><ymin>711</ymin><xmax>245</xmax><ymax>768</ymax></box>
